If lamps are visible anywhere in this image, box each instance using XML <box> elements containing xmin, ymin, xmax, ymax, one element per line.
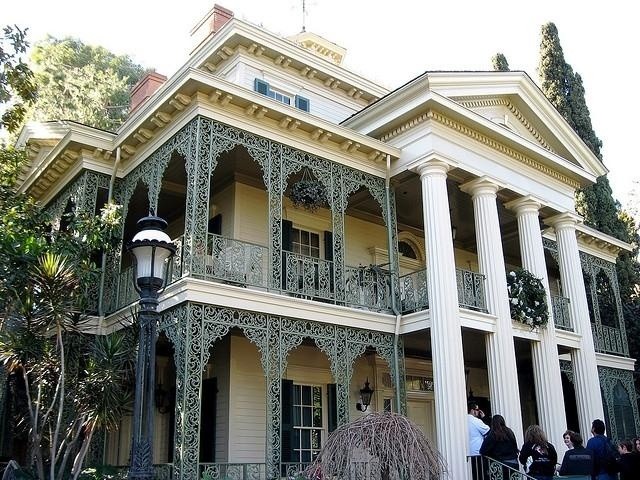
<box><xmin>155</xmin><ymin>380</ymin><xmax>171</xmax><ymax>414</ymax></box>
<box><xmin>356</xmin><ymin>376</ymin><xmax>374</xmax><ymax>411</ymax></box>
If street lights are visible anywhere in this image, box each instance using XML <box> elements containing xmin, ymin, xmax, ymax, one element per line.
<box><xmin>123</xmin><ymin>203</ymin><xmax>180</xmax><ymax>480</ymax></box>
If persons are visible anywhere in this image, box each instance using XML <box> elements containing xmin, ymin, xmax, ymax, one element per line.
<box><xmin>467</xmin><ymin>401</ymin><xmax>640</xmax><ymax>479</ymax></box>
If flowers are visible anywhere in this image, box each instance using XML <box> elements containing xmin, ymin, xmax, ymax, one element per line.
<box><xmin>507</xmin><ymin>267</ymin><xmax>550</xmax><ymax>334</ymax></box>
